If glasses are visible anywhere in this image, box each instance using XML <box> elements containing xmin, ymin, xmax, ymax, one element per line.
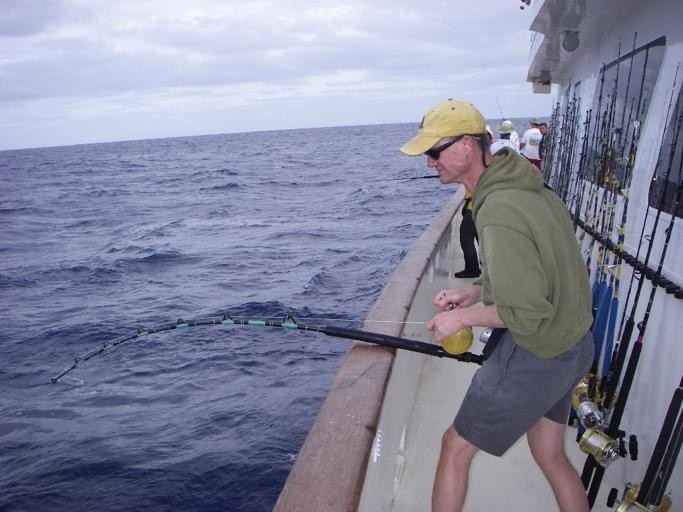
<box><xmin>426</xmin><ymin>139</ymin><xmax>466</xmax><ymax>160</ymax></box>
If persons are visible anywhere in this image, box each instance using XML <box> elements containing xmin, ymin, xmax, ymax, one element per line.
<box><xmin>398</xmin><ymin>98</ymin><xmax>595</xmax><ymax>512</ymax></box>
<box><xmin>454</xmin><ymin>115</ymin><xmax>548</xmax><ymax>277</ymax></box>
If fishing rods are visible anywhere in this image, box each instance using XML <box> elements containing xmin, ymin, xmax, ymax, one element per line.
<box><xmin>537</xmin><ymin>33</ymin><xmax>681</xmax><ymax>439</ymax></box>
<box><xmin>642</xmin><ymin>410</ymin><xmax>682</xmax><ymax>511</ymax></box>
<box><xmin>49</xmin><ymin>315</ymin><xmax>490</xmax><ymax>385</ymax></box>
<box><xmin>580</xmin><ymin>95</ymin><xmax>683</xmax><ymax>490</ymax></box>
<box><xmin>607</xmin><ymin>376</ymin><xmax>683</xmax><ymax>511</ymax></box>
<box><xmin>577</xmin><ymin>161</ymin><xmax>682</xmax><ymax>511</ymax></box>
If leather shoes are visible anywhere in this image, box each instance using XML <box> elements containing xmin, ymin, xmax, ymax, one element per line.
<box><xmin>455</xmin><ymin>268</ymin><xmax>480</xmax><ymax>278</ymax></box>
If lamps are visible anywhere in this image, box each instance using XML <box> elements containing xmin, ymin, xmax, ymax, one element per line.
<box><xmin>563</xmin><ymin>31</ymin><xmax>582</xmax><ymax>51</ymax></box>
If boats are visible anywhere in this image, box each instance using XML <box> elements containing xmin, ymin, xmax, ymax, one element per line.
<box><xmin>263</xmin><ymin>0</ymin><xmax>683</xmax><ymax>512</ymax></box>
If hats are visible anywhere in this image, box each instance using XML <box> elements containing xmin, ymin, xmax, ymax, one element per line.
<box><xmin>398</xmin><ymin>95</ymin><xmax>487</xmax><ymax>157</ymax></box>
<box><xmin>529</xmin><ymin>117</ymin><xmax>541</xmax><ymax>125</ymax></box>
<box><xmin>498</xmin><ymin>120</ymin><xmax>514</xmax><ymax>135</ymax></box>
